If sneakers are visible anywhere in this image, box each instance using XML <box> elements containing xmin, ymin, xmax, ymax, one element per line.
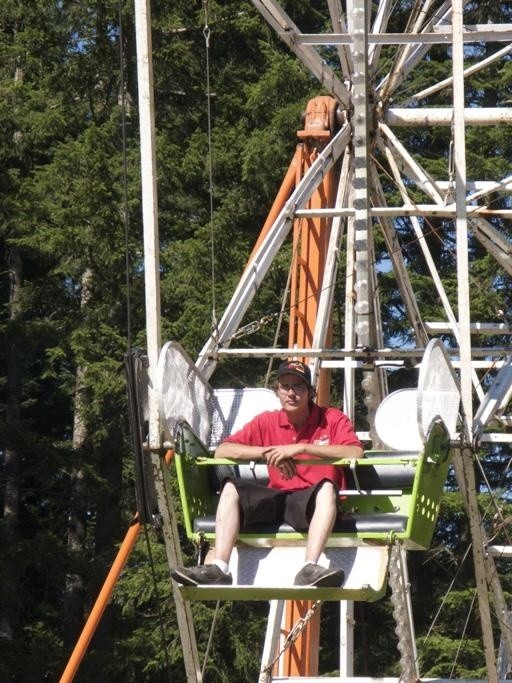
<box><xmin>171</xmin><ymin>564</ymin><xmax>232</xmax><ymax>585</ymax></box>
<box><xmin>294</xmin><ymin>563</ymin><xmax>344</xmax><ymax>586</ymax></box>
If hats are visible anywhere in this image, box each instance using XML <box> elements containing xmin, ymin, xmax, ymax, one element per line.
<box><xmin>277</xmin><ymin>361</ymin><xmax>311</xmax><ymax>387</ymax></box>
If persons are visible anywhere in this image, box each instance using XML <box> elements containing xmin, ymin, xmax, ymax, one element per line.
<box><xmin>169</xmin><ymin>360</ymin><xmax>366</xmax><ymax>585</ymax></box>
